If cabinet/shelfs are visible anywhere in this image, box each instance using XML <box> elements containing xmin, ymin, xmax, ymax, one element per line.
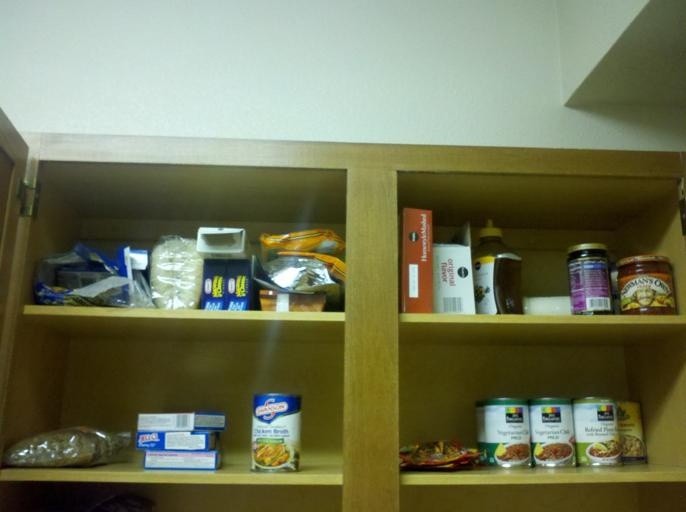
<box><xmin>0</xmin><ymin>109</ymin><xmax>686</xmax><ymax>512</ymax></box>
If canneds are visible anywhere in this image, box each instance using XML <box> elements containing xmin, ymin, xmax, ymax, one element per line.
<box><xmin>615</xmin><ymin>254</ymin><xmax>677</xmax><ymax>314</ymax></box>
<box><xmin>476</xmin><ymin>397</ymin><xmax>646</xmax><ymax>467</ymax></box>
<box><xmin>251</xmin><ymin>394</ymin><xmax>302</xmax><ymax>473</ymax></box>
<box><xmin>565</xmin><ymin>242</ymin><xmax>615</xmax><ymax>314</ymax></box>
